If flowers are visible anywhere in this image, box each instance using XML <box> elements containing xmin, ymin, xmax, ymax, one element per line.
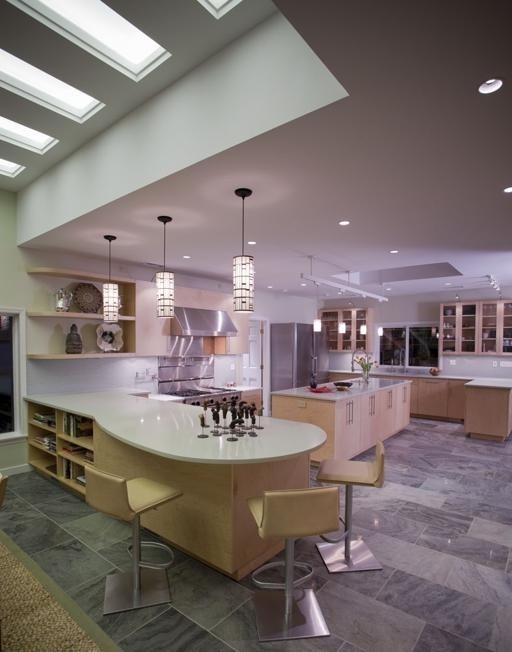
<box><xmin>352</xmin><ymin>348</ymin><xmax>380</xmax><ymax>376</ymax></box>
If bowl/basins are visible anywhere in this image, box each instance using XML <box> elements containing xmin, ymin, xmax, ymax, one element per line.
<box><xmin>336</xmin><ymin>386</ymin><xmax>349</xmax><ymax>391</ymax></box>
<box><xmin>444</xmin><ymin>309</ymin><xmax>454</xmax><ymax>315</ymax></box>
<box><xmin>333</xmin><ymin>382</ymin><xmax>354</xmax><ymax>387</ymax></box>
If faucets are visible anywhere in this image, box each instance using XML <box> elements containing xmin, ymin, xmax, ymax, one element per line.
<box><xmin>398</xmin><ymin>348</ymin><xmax>407</xmax><ymax>366</ymax></box>
<box><xmin>351</xmin><ymin>349</ymin><xmax>366</xmax><ymax>372</ymax></box>
<box><xmin>153</xmin><ymin>377</ymin><xmax>164</xmax><ymax>384</ymax></box>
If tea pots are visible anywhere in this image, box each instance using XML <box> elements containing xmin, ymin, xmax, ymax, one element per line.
<box><xmin>53</xmin><ymin>288</ymin><xmax>73</xmax><ymax>312</ymax></box>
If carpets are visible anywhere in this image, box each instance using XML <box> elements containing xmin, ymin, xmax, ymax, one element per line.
<box><xmin>1</xmin><ymin>531</ymin><xmax>126</xmax><ymax>652</ymax></box>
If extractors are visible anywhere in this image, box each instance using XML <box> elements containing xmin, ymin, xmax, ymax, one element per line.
<box><xmin>169</xmin><ymin>306</ymin><xmax>240</xmax><ymax>338</ymax></box>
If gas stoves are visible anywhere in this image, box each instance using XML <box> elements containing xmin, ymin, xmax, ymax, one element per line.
<box><xmin>164</xmin><ymin>386</ymin><xmax>242</xmax><ymax>405</ymax></box>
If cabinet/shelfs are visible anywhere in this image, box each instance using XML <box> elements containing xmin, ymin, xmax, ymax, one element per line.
<box><xmin>411</xmin><ymin>377</ymin><xmax>468</xmax><ymax>421</ymax></box>
<box><xmin>437</xmin><ymin>299</ymin><xmax>512</xmax><ymax>357</ymax></box>
<box><xmin>463</xmin><ymin>385</ymin><xmax>509</xmax><ymax>444</ymax></box>
<box><xmin>317</xmin><ymin>308</ymin><xmax>367</xmax><ymax>354</ymax></box>
<box><xmin>269</xmin><ymin>391</ymin><xmax>362</xmax><ymax>465</ymax></box>
<box><xmin>359</xmin><ymin>388</ymin><xmax>378</xmax><ymax>453</ymax></box>
<box><xmin>378</xmin><ymin>383</ymin><xmax>397</xmax><ymax>443</ymax></box>
<box><xmin>396</xmin><ymin>380</ymin><xmax>411</xmax><ymax>435</ymax></box>
<box><xmin>213</xmin><ymin>310</ymin><xmax>250</xmax><ymax>355</ymax></box>
<box><xmin>25</xmin><ymin>267</ymin><xmax>140</xmax><ymax>362</ymax></box>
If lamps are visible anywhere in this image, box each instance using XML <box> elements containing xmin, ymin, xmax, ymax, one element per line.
<box><xmin>230</xmin><ymin>187</ymin><xmax>257</xmax><ymax>314</ymax></box>
<box><xmin>154</xmin><ymin>216</ymin><xmax>176</xmax><ymax>320</ymax></box>
<box><xmin>299</xmin><ymin>255</ymin><xmax>389</xmax><ymax>337</ymax></box>
<box><xmin>101</xmin><ymin>233</ymin><xmax>122</xmax><ymax>325</ymax></box>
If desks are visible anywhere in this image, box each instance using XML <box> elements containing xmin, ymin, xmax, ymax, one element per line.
<box><xmin>21</xmin><ymin>386</ymin><xmax>327</xmax><ymax>583</ymax></box>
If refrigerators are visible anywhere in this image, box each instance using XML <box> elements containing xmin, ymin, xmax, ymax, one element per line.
<box><xmin>269</xmin><ymin>322</ymin><xmax>330</xmax><ymax>393</ymax></box>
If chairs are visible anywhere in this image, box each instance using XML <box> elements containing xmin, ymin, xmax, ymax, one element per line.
<box><xmin>81</xmin><ymin>460</ymin><xmax>186</xmax><ymax>617</ymax></box>
<box><xmin>243</xmin><ymin>485</ymin><xmax>343</xmax><ymax>642</ymax></box>
<box><xmin>312</xmin><ymin>438</ymin><xmax>390</xmax><ymax>575</ymax></box>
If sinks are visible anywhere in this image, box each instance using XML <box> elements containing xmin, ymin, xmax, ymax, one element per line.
<box><xmin>148</xmin><ymin>393</ymin><xmax>184</xmax><ymax>403</ymax></box>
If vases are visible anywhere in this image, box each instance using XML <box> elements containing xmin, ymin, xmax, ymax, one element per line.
<box><xmin>360</xmin><ymin>367</ymin><xmax>370</xmax><ymax>381</ymax></box>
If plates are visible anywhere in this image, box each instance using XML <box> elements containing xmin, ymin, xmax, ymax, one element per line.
<box><xmin>96</xmin><ymin>323</ymin><xmax>124</xmax><ymax>352</ymax></box>
<box><xmin>72</xmin><ymin>282</ymin><xmax>102</xmax><ymax>314</ymax></box>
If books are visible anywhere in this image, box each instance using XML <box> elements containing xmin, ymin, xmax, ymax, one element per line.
<box><xmin>31</xmin><ymin>410</ymin><xmax>95</xmax><ymax>486</ymax></box>
